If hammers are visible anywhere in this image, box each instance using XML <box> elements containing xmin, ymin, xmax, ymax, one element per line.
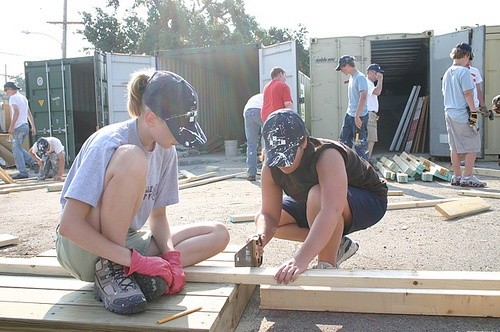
<box><xmin>488</xmin><ymin>109</ymin><xmax>494</xmax><ymax>120</ymax></box>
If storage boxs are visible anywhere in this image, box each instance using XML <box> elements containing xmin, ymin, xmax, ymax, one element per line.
<box><xmin>25</xmin><ymin>23</ymin><xmax>500</xmax><ymax>168</ymax></box>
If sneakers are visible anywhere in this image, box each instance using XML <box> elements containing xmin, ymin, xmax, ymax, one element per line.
<box><xmin>451</xmin><ymin>175</ymin><xmax>462</xmax><ymax>185</ymax></box>
<box><xmin>134</xmin><ymin>274</ymin><xmax>168</xmax><ymax>303</ymax></box>
<box><xmin>312</xmin><ymin>236</ymin><xmax>359</xmax><ymax>269</ymax></box>
<box><xmin>459</xmin><ymin>175</ymin><xmax>487</xmax><ymax>187</ymax></box>
<box><xmin>94</xmin><ymin>255</ymin><xmax>149</xmax><ymax>314</ymax></box>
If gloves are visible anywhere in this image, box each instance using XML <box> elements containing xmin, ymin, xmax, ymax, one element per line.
<box><xmin>123</xmin><ymin>248</ymin><xmax>173</xmax><ymax>287</ymax></box>
<box><xmin>162</xmin><ymin>250</ymin><xmax>186</xmax><ymax>294</ymax></box>
<box><xmin>481</xmin><ymin>106</ymin><xmax>491</xmax><ymax>118</ymax></box>
<box><xmin>468</xmin><ymin>112</ymin><xmax>478</xmax><ymax>124</ymax></box>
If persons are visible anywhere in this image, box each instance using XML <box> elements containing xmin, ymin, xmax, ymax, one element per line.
<box><xmin>335</xmin><ymin>55</ymin><xmax>369</xmax><ymax>162</ymax></box>
<box><xmin>246</xmin><ymin>108</ymin><xmax>388</xmax><ymax>285</ymax></box>
<box><xmin>29</xmin><ymin>137</ymin><xmax>65</xmax><ymax>182</ymax></box>
<box><xmin>261</xmin><ymin>67</ymin><xmax>294</xmax><ymax>123</ymax></box>
<box><xmin>56</xmin><ymin>71</ymin><xmax>230</xmax><ymax>314</ymax></box>
<box><xmin>243</xmin><ymin>93</ymin><xmax>263</xmax><ymax>180</ymax></box>
<box><xmin>441</xmin><ymin>42</ymin><xmax>488</xmax><ymax>187</ymax></box>
<box><xmin>364</xmin><ymin>64</ymin><xmax>384</xmax><ymax>157</ymax></box>
<box><xmin>4</xmin><ymin>82</ymin><xmax>40</xmax><ymax>179</ymax></box>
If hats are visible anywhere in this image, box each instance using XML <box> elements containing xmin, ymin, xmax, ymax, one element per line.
<box><xmin>37</xmin><ymin>138</ymin><xmax>48</xmax><ymax>154</ymax></box>
<box><xmin>262</xmin><ymin>108</ymin><xmax>310</xmax><ymax>168</ymax></box>
<box><xmin>4</xmin><ymin>82</ymin><xmax>20</xmax><ymax>90</ymax></box>
<box><xmin>367</xmin><ymin>64</ymin><xmax>384</xmax><ymax>73</ymax></box>
<box><xmin>457</xmin><ymin>43</ymin><xmax>473</xmax><ymax>60</ymax></box>
<box><xmin>144</xmin><ymin>70</ymin><xmax>208</xmax><ymax>149</ymax></box>
<box><xmin>334</xmin><ymin>55</ymin><xmax>355</xmax><ymax>71</ymax></box>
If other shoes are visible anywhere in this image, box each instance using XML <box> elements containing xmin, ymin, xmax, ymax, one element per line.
<box><xmin>247</xmin><ymin>176</ymin><xmax>256</xmax><ymax>181</ymax></box>
<box><xmin>368</xmin><ymin>160</ymin><xmax>379</xmax><ymax>171</ymax></box>
<box><xmin>34</xmin><ymin>162</ymin><xmax>40</xmax><ymax>174</ymax></box>
<box><xmin>12</xmin><ymin>174</ymin><xmax>29</xmax><ymax>179</ymax></box>
<box><xmin>460</xmin><ymin>160</ymin><xmax>465</xmax><ymax>166</ymax></box>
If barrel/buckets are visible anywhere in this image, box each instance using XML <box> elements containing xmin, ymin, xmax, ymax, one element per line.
<box><xmin>224</xmin><ymin>140</ymin><xmax>238</xmax><ymax>157</ymax></box>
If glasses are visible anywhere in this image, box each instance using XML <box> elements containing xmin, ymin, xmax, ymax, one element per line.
<box><xmin>4</xmin><ymin>89</ymin><xmax>10</xmax><ymax>92</ymax></box>
